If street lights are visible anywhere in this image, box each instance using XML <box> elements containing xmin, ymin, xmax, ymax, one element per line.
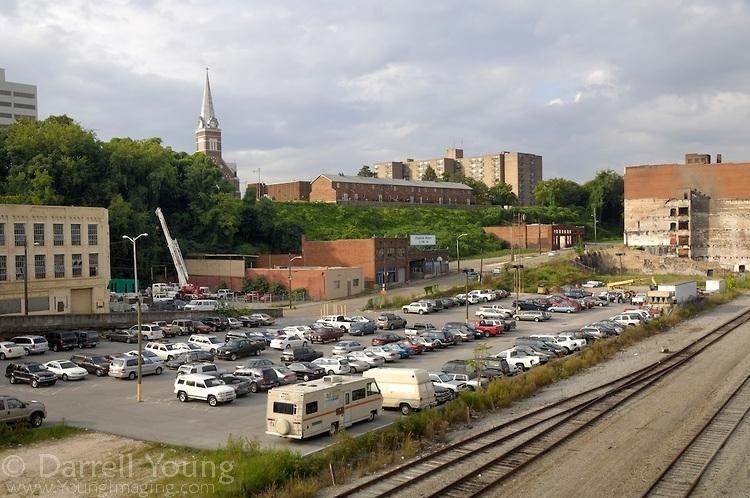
<box><xmin>252</xmin><ymin>166</ymin><xmax>261</xmax><ymax>200</ymax></box>
<box><xmin>460</xmin><ymin>267</ymin><xmax>475</xmax><ymax>322</ymax></box>
<box><xmin>290</xmin><ymin>254</ymin><xmax>304</xmax><ymax>309</ymax></box>
<box><xmin>456</xmin><ymin>232</ymin><xmax>470</xmax><ymax>272</ymax></box>
<box><xmin>121</xmin><ymin>231</ymin><xmax>150</xmax><ymax>295</ymax></box>
<box><xmin>512</xmin><ymin>262</ymin><xmax>525</xmax><ymax>314</ymax></box>
<box><xmin>23</xmin><ymin>240</ymin><xmax>41</xmax><ymax>314</ymax></box>
<box><xmin>614</xmin><ymin>252</ymin><xmax>626</xmax><ymax>278</ymax></box>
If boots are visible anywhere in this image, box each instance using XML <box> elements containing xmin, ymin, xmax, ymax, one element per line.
<box><xmin>0</xmin><ymin>393</ymin><xmax>47</xmax><ymax>429</ymax></box>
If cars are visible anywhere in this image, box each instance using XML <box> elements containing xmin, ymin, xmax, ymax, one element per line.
<box><xmin>0</xmin><ymin>277</ymin><xmax>662</xmax><ymax>405</ymax></box>
<box><xmin>493</xmin><ymin>265</ymin><xmax>503</xmax><ymax>273</ymax></box>
<box><xmin>548</xmin><ymin>250</ymin><xmax>562</xmax><ymax>257</ymax></box>
<box><xmin>466</xmin><ymin>271</ymin><xmax>479</xmax><ymax>281</ymax></box>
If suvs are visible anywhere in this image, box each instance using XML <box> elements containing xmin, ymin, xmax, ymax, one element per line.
<box><xmin>0</xmin><ymin>393</ymin><xmax>47</xmax><ymax>429</ymax></box>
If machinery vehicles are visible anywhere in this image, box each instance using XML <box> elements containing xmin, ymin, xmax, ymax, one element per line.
<box><xmin>154</xmin><ymin>206</ymin><xmax>218</xmax><ymax>300</ymax></box>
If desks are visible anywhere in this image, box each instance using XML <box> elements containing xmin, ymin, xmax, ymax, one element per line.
<box><xmin>456</xmin><ymin>232</ymin><xmax>470</xmax><ymax>272</ymax></box>
<box><xmin>512</xmin><ymin>262</ymin><xmax>525</xmax><ymax>314</ymax></box>
<box><xmin>23</xmin><ymin>240</ymin><xmax>41</xmax><ymax>314</ymax></box>
<box><xmin>252</xmin><ymin>166</ymin><xmax>261</xmax><ymax>200</ymax></box>
<box><xmin>290</xmin><ymin>254</ymin><xmax>304</xmax><ymax>309</ymax></box>
<box><xmin>121</xmin><ymin>231</ymin><xmax>150</xmax><ymax>295</ymax></box>
<box><xmin>460</xmin><ymin>267</ymin><xmax>475</xmax><ymax>322</ymax></box>
<box><xmin>614</xmin><ymin>252</ymin><xmax>626</xmax><ymax>278</ymax></box>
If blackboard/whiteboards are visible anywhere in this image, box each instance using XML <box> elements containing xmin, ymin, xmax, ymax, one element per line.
<box><xmin>154</xmin><ymin>206</ymin><xmax>218</xmax><ymax>300</ymax></box>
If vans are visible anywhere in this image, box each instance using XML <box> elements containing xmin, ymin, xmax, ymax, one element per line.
<box><xmin>360</xmin><ymin>366</ymin><xmax>437</xmax><ymax>415</ymax></box>
<box><xmin>261</xmin><ymin>370</ymin><xmax>386</xmax><ymax>443</ymax></box>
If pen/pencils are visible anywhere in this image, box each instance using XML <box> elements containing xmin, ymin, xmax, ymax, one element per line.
<box><xmin>261</xmin><ymin>370</ymin><xmax>386</xmax><ymax>443</ymax></box>
<box><xmin>360</xmin><ymin>366</ymin><xmax>437</xmax><ymax>415</ymax></box>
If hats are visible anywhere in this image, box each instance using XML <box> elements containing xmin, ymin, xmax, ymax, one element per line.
<box><xmin>493</xmin><ymin>265</ymin><xmax>503</xmax><ymax>273</ymax></box>
<box><xmin>548</xmin><ymin>250</ymin><xmax>562</xmax><ymax>257</ymax></box>
<box><xmin>466</xmin><ymin>271</ymin><xmax>479</xmax><ymax>281</ymax></box>
